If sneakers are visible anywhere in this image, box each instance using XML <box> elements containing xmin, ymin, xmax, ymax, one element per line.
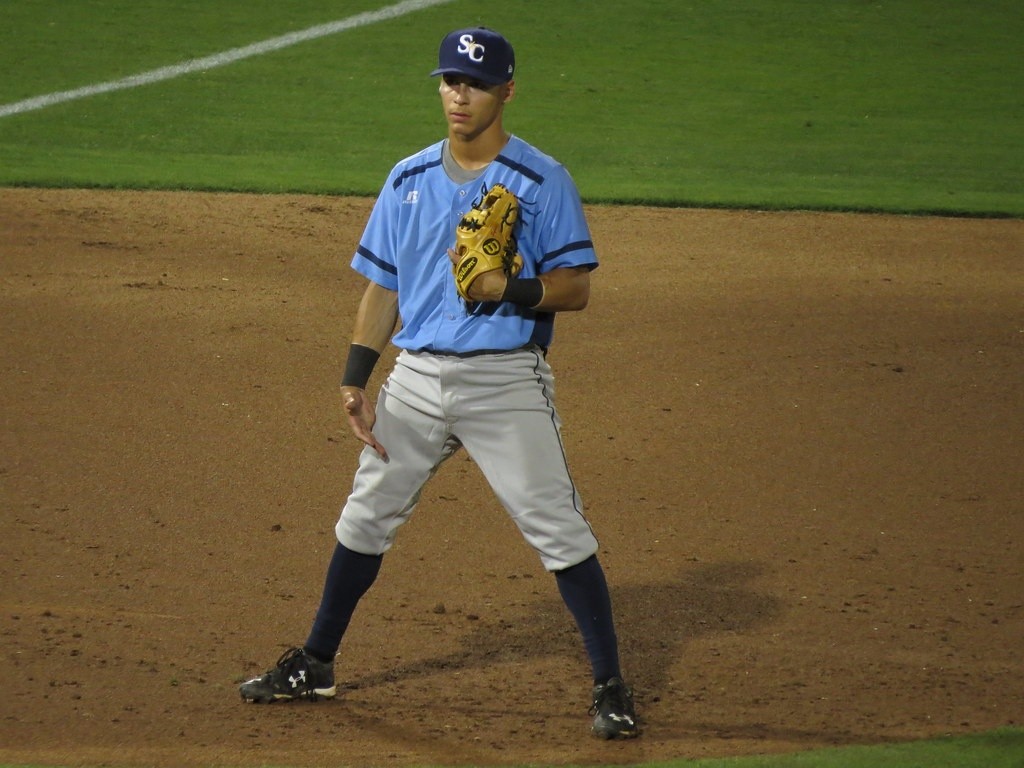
<box><xmin>587</xmin><ymin>677</ymin><xmax>637</xmax><ymax>739</ymax></box>
<box><xmin>237</xmin><ymin>646</ymin><xmax>336</xmax><ymax>704</ymax></box>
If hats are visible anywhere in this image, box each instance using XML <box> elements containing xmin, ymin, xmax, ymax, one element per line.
<box><xmin>430</xmin><ymin>26</ymin><xmax>516</xmax><ymax>84</ymax></box>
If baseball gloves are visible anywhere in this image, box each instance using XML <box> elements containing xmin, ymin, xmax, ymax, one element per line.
<box><xmin>450</xmin><ymin>178</ymin><xmax>524</xmax><ymax>305</ymax></box>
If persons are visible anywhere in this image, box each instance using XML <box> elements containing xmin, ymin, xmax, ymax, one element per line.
<box><xmin>236</xmin><ymin>27</ymin><xmax>644</xmax><ymax>740</ymax></box>
